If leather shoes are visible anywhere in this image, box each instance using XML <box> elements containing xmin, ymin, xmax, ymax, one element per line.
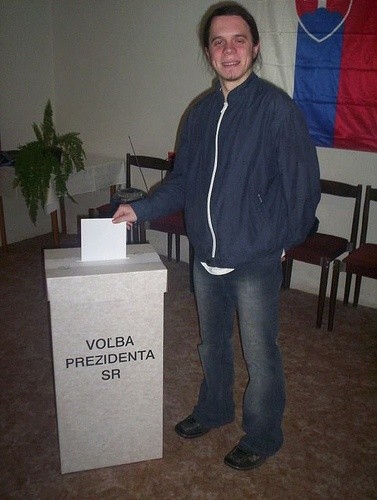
<box><xmin>175</xmin><ymin>413</ymin><xmax>212</xmax><ymax>439</ymax></box>
<box><xmin>223</xmin><ymin>442</ymin><xmax>268</xmax><ymax>470</ymax></box>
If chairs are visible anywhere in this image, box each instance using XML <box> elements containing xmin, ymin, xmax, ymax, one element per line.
<box><xmin>88</xmin><ymin>153</ymin><xmax>194</xmax><ymax>293</ymax></box>
<box><xmin>280</xmin><ymin>179</ymin><xmax>377</xmax><ymax>333</ymax></box>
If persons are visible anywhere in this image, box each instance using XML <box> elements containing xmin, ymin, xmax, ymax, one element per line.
<box><xmin>113</xmin><ymin>5</ymin><xmax>321</xmax><ymax>471</ymax></box>
